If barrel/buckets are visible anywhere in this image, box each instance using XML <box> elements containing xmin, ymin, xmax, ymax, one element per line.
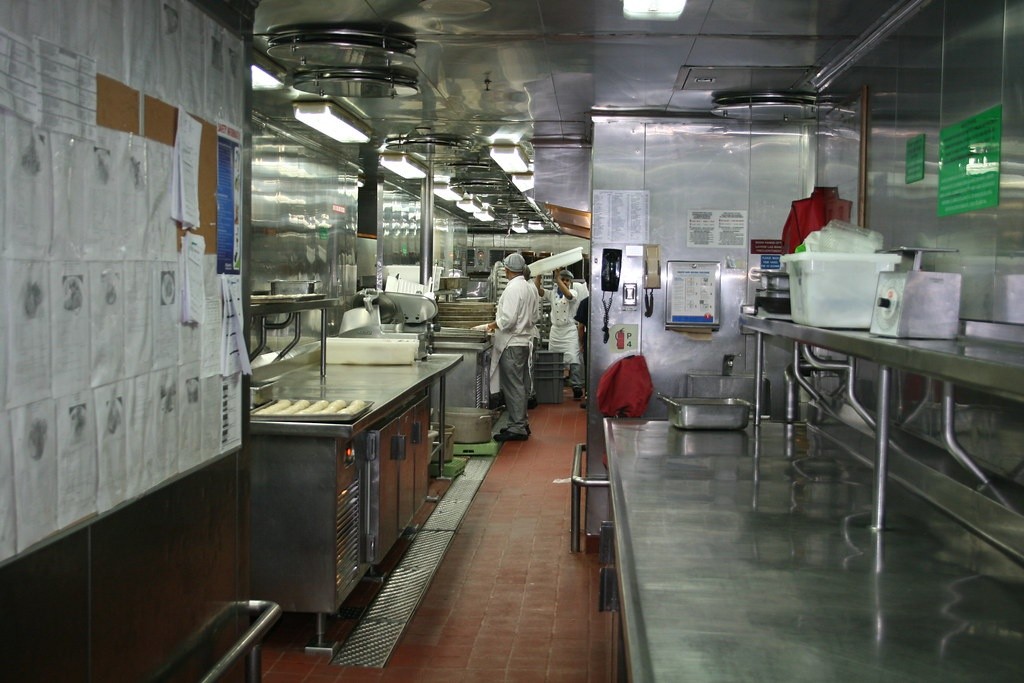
<box><xmin>268</xmin><ymin>279</ymin><xmax>321</xmax><ymax>294</ymax></box>
<box><xmin>657</xmin><ymin>393</ymin><xmax>751</xmax><ymax>429</ymax></box>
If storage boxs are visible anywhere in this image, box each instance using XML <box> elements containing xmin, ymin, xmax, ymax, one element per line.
<box><xmin>533</xmin><ymin>350</ymin><xmax>567</xmax><ymax>404</ymax></box>
<box><xmin>780</xmin><ymin>251</ymin><xmax>903</xmax><ymax>330</ymax></box>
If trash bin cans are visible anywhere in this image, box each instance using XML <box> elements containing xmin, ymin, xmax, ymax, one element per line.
<box><xmin>533</xmin><ymin>350</ymin><xmax>564</xmax><ymax>404</ymax></box>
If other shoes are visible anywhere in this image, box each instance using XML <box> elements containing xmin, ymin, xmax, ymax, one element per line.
<box><xmin>573</xmin><ymin>387</ymin><xmax>583</xmax><ymax>399</ymax></box>
<box><xmin>527</xmin><ymin>399</ymin><xmax>537</xmax><ymax>409</ymax></box>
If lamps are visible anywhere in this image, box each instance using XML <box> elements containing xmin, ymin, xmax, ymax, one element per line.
<box><xmin>251</xmin><ymin>0</ymin><xmax>688</xmax><ymax>236</ymax></box>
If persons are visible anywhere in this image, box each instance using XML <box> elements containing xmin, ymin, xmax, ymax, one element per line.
<box><xmin>574</xmin><ymin>263</ymin><xmax>589</xmax><ymax>334</ymax></box>
<box><xmin>468</xmin><ymin>252</ymin><xmax>531</xmax><ymax>442</ymax></box>
<box><xmin>574</xmin><ymin>272</ymin><xmax>589</xmax><ymax>402</ymax></box>
<box><xmin>520</xmin><ymin>263</ymin><xmax>541</xmax><ymax>410</ymax></box>
<box><xmin>534</xmin><ymin>266</ymin><xmax>584</xmax><ymax>401</ymax></box>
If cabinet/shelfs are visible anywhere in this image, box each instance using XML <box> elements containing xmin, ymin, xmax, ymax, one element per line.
<box><xmin>379</xmin><ymin>397</ymin><xmax>430</xmax><ymax>561</ymax></box>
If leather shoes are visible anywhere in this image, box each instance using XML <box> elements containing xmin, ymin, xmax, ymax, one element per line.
<box><xmin>500</xmin><ymin>425</ymin><xmax>531</xmax><ymax>435</ymax></box>
<box><xmin>494</xmin><ymin>431</ymin><xmax>527</xmax><ymax>441</ymax></box>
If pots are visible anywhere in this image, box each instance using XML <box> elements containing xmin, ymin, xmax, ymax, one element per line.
<box><xmin>446</xmin><ymin>406</ymin><xmax>499</xmax><ymax>444</ymax></box>
<box><xmin>433</xmin><ymin>422</ymin><xmax>454</xmax><ymax>464</ymax></box>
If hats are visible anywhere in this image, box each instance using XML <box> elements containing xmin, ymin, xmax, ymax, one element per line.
<box><xmin>505</xmin><ymin>253</ymin><xmax>526</xmax><ymax>272</ymax></box>
<box><xmin>559</xmin><ymin>270</ymin><xmax>574</xmax><ymax>278</ymax></box>
